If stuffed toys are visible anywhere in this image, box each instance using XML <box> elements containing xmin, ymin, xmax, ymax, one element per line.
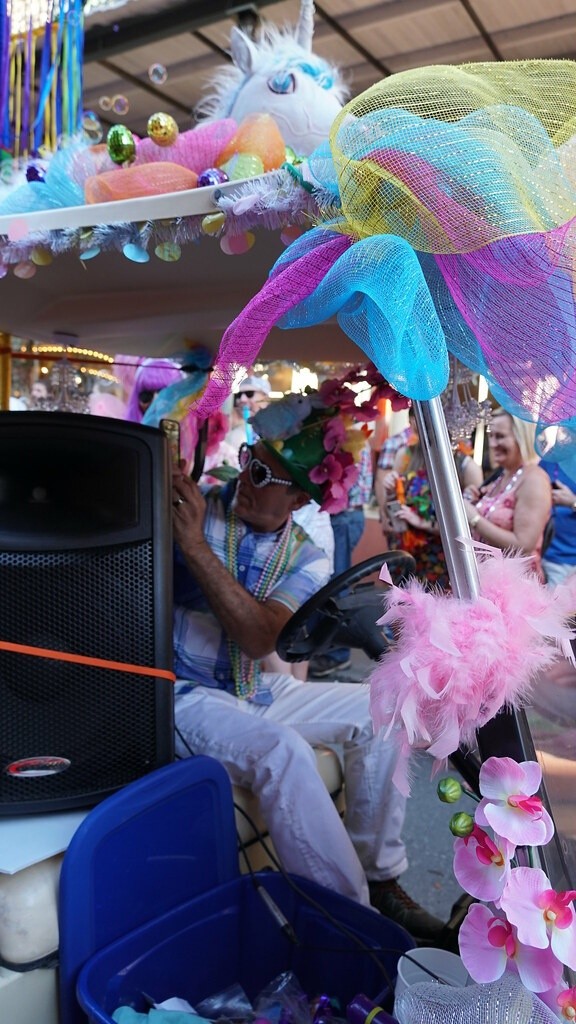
<box><xmin>194</xmin><ymin>0</ymin><xmax>384</xmax><ymax>160</ymax></box>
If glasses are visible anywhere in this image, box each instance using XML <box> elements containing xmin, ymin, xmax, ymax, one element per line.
<box><xmin>235</xmin><ymin>390</ymin><xmax>261</xmax><ymax>398</ymax></box>
<box><xmin>138</xmin><ymin>387</ymin><xmax>175</xmax><ymax>403</ymax></box>
<box><xmin>237</xmin><ymin>442</ymin><xmax>293</xmax><ymax>489</ymax></box>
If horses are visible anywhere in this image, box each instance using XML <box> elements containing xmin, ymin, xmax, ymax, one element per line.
<box><xmin>192</xmin><ymin>0</ymin><xmax>356</xmax><ymax>156</ymax></box>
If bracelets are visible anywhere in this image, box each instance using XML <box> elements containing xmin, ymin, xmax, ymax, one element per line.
<box><xmin>470</xmin><ymin>515</ymin><xmax>480</xmax><ymax>528</ymax></box>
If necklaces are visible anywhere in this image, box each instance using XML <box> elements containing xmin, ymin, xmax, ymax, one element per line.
<box><xmin>227</xmin><ymin>503</ymin><xmax>294</xmax><ymax>700</ymax></box>
<box><xmin>476</xmin><ymin>462</ymin><xmax>523</xmax><ymax>518</ymax></box>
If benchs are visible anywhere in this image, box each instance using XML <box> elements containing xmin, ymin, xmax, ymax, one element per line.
<box><xmin>0</xmin><ymin>750</ymin><xmax>342</xmax><ymax>968</ymax></box>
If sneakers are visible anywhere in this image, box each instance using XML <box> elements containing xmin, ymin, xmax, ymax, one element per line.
<box><xmin>367</xmin><ymin>875</ymin><xmax>447</xmax><ymax>942</ymax></box>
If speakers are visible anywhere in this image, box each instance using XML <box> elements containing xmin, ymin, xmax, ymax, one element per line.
<box><xmin>0</xmin><ymin>410</ymin><xmax>180</xmax><ymax>817</ymax></box>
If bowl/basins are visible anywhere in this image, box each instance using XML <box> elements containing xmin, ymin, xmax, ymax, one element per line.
<box><xmin>393</xmin><ymin>947</ymin><xmax>469</xmax><ymax>1021</ymax></box>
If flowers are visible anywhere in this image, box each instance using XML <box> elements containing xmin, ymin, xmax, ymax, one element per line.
<box><xmin>438</xmin><ymin>757</ymin><xmax>576</xmax><ymax>1023</ymax></box>
<box><xmin>309</xmin><ymin>362</ymin><xmax>413</xmax><ymax>515</ymax></box>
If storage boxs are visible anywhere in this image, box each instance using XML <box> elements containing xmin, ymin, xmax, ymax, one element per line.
<box><xmin>55</xmin><ymin>754</ymin><xmax>415</xmax><ymax>1023</ymax></box>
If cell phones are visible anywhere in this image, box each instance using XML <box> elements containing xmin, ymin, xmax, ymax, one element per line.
<box><xmin>157</xmin><ymin>417</ymin><xmax>181</xmax><ymax>475</ymax></box>
<box><xmin>385</xmin><ymin>501</ymin><xmax>408</xmax><ymax>533</ymax></box>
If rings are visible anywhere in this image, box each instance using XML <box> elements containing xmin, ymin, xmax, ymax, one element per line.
<box><xmin>173</xmin><ymin>497</ymin><xmax>184</xmax><ymax>507</ymax></box>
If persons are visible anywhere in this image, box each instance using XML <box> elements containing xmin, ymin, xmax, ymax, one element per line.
<box><xmin>375</xmin><ymin>402</ymin><xmax>576</xmax><ymax>789</ymax></box>
<box><xmin>172</xmin><ymin>397</ymin><xmax>461</xmax><ymax>957</ymax></box>
<box><xmin>309</xmin><ymin>413</ymin><xmax>373</xmax><ymax>677</ymax></box>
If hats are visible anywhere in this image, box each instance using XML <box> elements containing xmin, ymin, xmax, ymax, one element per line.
<box><xmin>245</xmin><ymin>363</ymin><xmax>412</xmax><ymax>514</ymax></box>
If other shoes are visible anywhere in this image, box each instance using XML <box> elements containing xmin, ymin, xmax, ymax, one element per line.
<box><xmin>308</xmin><ymin>655</ymin><xmax>350</xmax><ymax>676</ymax></box>
